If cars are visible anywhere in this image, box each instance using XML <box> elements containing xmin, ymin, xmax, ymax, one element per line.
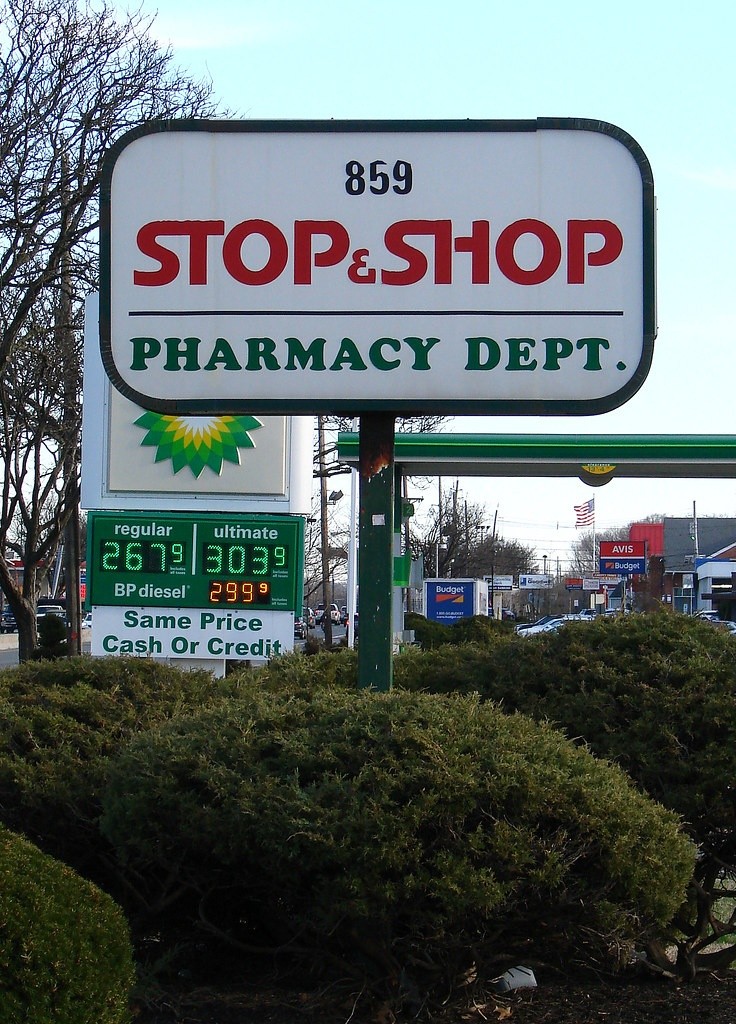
<box><xmin>340</xmin><ymin>606</ymin><xmax>347</xmax><ymax>624</ymax></box>
<box><xmin>1</xmin><ymin>604</ymin><xmax>93</xmax><ymax>634</ymax></box>
<box><xmin>516</xmin><ymin>607</ymin><xmax>630</xmax><ymax>637</ymax></box>
<box><xmin>303</xmin><ymin>607</ymin><xmax>315</xmax><ymax>629</ymax></box>
<box><xmin>294</xmin><ymin>621</ymin><xmax>307</xmax><ymax>639</ymax></box>
<box><xmin>489</xmin><ymin>608</ymin><xmax>515</xmax><ymax>622</ymax></box>
<box><xmin>691</xmin><ymin>610</ymin><xmax>736</xmax><ymax>634</ymax></box>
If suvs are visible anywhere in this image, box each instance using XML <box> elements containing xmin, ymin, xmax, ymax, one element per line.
<box><xmin>315</xmin><ymin>604</ymin><xmax>340</xmax><ymax>625</ymax></box>
